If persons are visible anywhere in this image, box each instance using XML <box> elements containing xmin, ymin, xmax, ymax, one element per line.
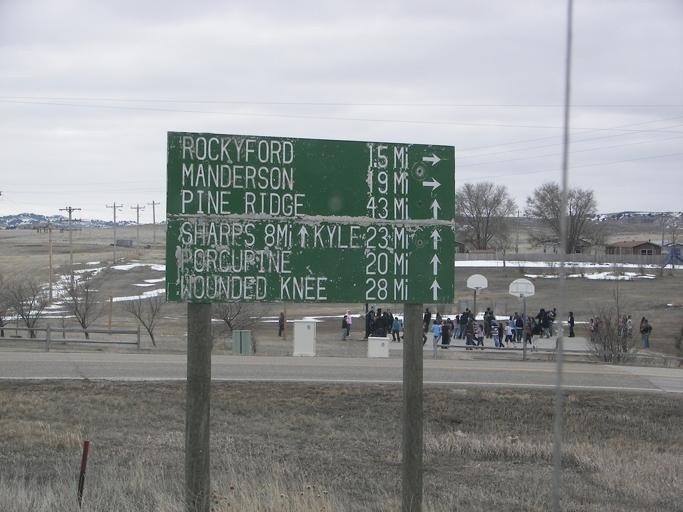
<box><xmin>340</xmin><ymin>305</ymin><xmax>633</xmax><ymax>351</ymax></box>
<box><xmin>641</xmin><ymin>319</ymin><xmax>652</xmax><ymax>348</ymax></box>
<box><xmin>639</xmin><ymin>317</ymin><xmax>646</xmax><ymax>334</ymax></box>
<box><xmin>278</xmin><ymin>312</ymin><xmax>283</xmax><ymax>336</ymax></box>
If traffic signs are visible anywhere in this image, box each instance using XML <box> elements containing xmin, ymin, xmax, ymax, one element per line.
<box><xmin>163</xmin><ymin>131</ymin><xmax>458</xmax><ymax>305</ymax></box>
